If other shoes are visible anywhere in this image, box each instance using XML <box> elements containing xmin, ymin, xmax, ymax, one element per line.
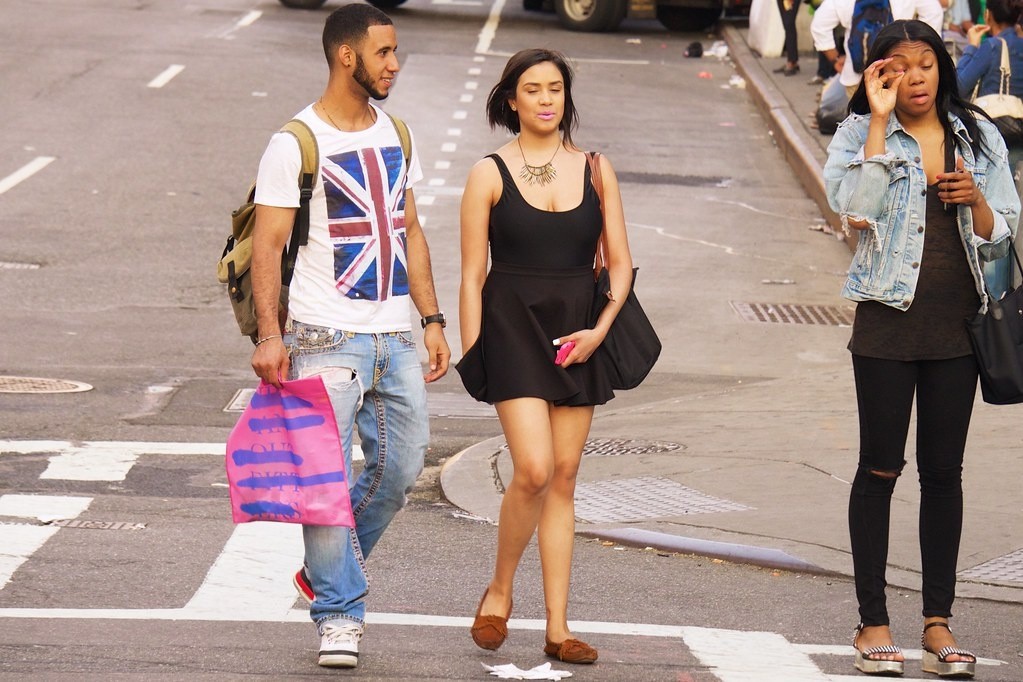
<box><xmin>471</xmin><ymin>587</ymin><xmax>514</xmax><ymax>650</ymax></box>
<box><xmin>543</xmin><ymin>634</ymin><xmax>598</xmax><ymax>664</ymax></box>
<box><xmin>773</xmin><ymin>62</ymin><xmax>800</xmax><ymax>76</ymax></box>
<box><xmin>806</xmin><ymin>76</ymin><xmax>824</xmax><ymax>86</ymax></box>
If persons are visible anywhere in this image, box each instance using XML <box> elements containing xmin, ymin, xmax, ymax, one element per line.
<box><xmin>453</xmin><ymin>48</ymin><xmax>633</xmax><ymax>665</ymax></box>
<box><xmin>772</xmin><ymin>0</ymin><xmax>800</xmax><ymax>76</ymax></box>
<box><xmin>804</xmin><ymin>0</ymin><xmax>944</xmax><ymax>134</ymax></box>
<box><xmin>250</xmin><ymin>2</ymin><xmax>450</xmax><ymax>666</ymax></box>
<box><xmin>824</xmin><ymin>19</ymin><xmax>1021</xmax><ymax>681</ymax></box>
<box><xmin>942</xmin><ymin>0</ymin><xmax>973</xmax><ymax>38</ymax></box>
<box><xmin>955</xmin><ymin>0</ymin><xmax>1023</xmax><ymax>300</ymax></box>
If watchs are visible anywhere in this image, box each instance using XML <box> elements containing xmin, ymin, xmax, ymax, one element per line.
<box><xmin>421</xmin><ymin>312</ymin><xmax>446</xmax><ymax>328</ymax></box>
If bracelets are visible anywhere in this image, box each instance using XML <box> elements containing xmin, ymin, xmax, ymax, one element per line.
<box><xmin>256</xmin><ymin>335</ymin><xmax>283</xmax><ymax>345</ymax></box>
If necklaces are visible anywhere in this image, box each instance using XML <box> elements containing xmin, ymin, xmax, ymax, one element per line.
<box><xmin>321</xmin><ymin>97</ymin><xmax>375</xmax><ymax>131</ymax></box>
<box><xmin>518</xmin><ymin>137</ymin><xmax>561</xmax><ymax>187</ymax></box>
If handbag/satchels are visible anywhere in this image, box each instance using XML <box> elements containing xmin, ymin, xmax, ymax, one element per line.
<box><xmin>965</xmin><ymin>36</ymin><xmax>1023</xmax><ymax>147</ymax></box>
<box><xmin>226</xmin><ymin>371</ymin><xmax>357</xmax><ymax>527</ymax></box>
<box><xmin>965</xmin><ymin>212</ymin><xmax>1023</xmax><ymax>405</ymax></box>
<box><xmin>582</xmin><ymin>149</ymin><xmax>662</xmax><ymax>390</ymax></box>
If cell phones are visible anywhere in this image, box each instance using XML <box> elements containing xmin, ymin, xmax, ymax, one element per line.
<box><xmin>554</xmin><ymin>340</ymin><xmax>575</xmax><ymax>365</ymax></box>
<box><xmin>944</xmin><ymin>170</ymin><xmax>963</xmax><ymax>211</ymax></box>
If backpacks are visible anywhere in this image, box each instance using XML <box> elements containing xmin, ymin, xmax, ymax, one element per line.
<box><xmin>215</xmin><ymin>108</ymin><xmax>410</xmax><ymax>343</ymax></box>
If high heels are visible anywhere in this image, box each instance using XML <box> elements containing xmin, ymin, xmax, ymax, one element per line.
<box><xmin>920</xmin><ymin>621</ymin><xmax>979</xmax><ymax>678</ymax></box>
<box><xmin>853</xmin><ymin>622</ymin><xmax>904</xmax><ymax>676</ymax></box>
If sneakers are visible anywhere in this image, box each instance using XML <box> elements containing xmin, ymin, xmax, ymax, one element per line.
<box><xmin>293</xmin><ymin>565</ymin><xmax>318</xmax><ymax>606</ymax></box>
<box><xmin>314</xmin><ymin>618</ymin><xmax>362</xmax><ymax>669</ymax></box>
